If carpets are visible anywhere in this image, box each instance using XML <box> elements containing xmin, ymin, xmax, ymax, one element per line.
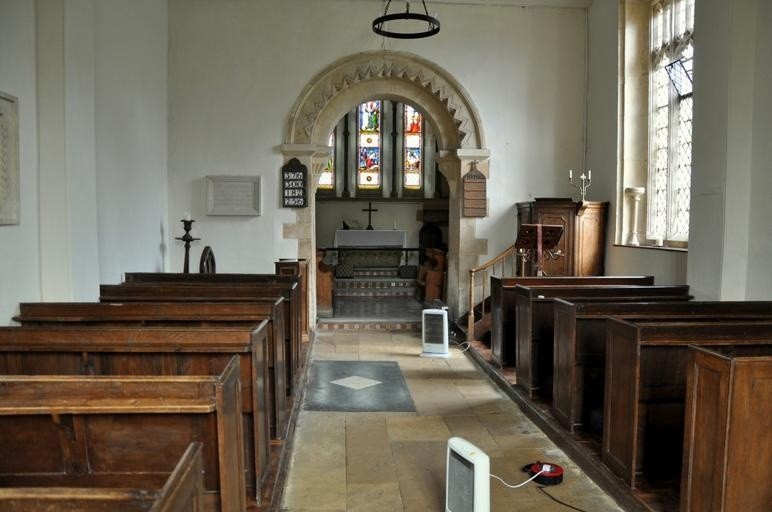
<box><xmin>304</xmin><ymin>361</ymin><xmax>417</xmax><ymax>412</ymax></box>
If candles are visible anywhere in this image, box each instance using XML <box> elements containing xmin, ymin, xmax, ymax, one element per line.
<box><xmin>567</xmin><ymin>170</ymin><xmax>592</xmax><ymax>201</ymax></box>
<box><xmin>174</xmin><ymin>211</ymin><xmax>202</xmax><ymax>273</ymax></box>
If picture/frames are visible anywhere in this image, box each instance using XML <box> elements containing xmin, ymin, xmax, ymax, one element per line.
<box><xmin>205</xmin><ymin>175</ymin><xmax>262</xmax><ymax>216</ymax></box>
<box><xmin>0</xmin><ymin>92</ymin><xmax>21</xmax><ymax>227</ymax></box>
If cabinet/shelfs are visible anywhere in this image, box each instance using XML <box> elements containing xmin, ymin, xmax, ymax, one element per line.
<box><xmin>513</xmin><ymin>196</ymin><xmax>610</xmax><ymax>276</ymax></box>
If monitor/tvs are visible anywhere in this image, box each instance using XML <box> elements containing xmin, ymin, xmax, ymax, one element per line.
<box><xmin>446</xmin><ymin>438</ymin><xmax>490</xmax><ymax>512</ymax></box>
<box><xmin>421</xmin><ymin>310</ymin><xmax>450</xmax><ymax>359</ymax></box>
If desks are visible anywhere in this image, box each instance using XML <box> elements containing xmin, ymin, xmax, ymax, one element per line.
<box><xmin>0</xmin><ymin>273</ymin><xmax>303</xmax><ymax>512</ymax></box>
<box><xmin>490</xmin><ymin>275</ymin><xmax>772</xmax><ymax>511</ymax></box>
